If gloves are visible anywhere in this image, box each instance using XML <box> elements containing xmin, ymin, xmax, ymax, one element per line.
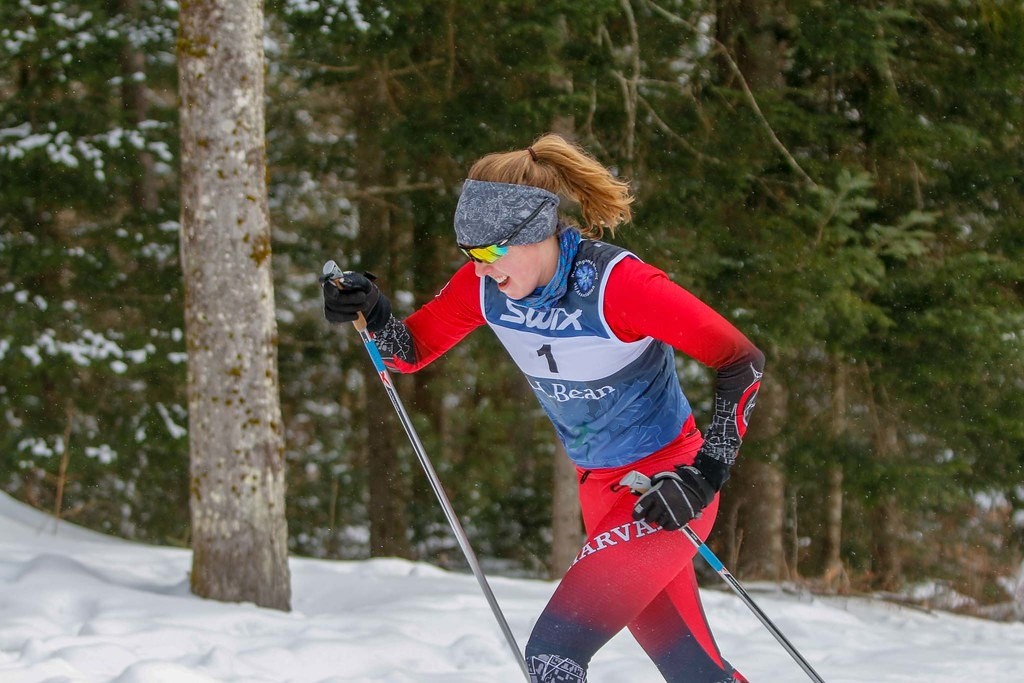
<box><xmin>319</xmin><ymin>270</ymin><xmax>392</xmax><ymax>333</ymax></box>
<box><xmin>631</xmin><ymin>464</ymin><xmax>715</xmax><ymax>532</ymax></box>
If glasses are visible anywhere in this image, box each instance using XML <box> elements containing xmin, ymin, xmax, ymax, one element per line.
<box><xmin>458</xmin><ymin>200</ymin><xmax>549</xmax><ymax>264</ymax></box>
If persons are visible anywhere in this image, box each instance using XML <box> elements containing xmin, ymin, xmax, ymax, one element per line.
<box><xmin>322</xmin><ymin>134</ymin><xmax>765</xmax><ymax>683</ymax></box>
